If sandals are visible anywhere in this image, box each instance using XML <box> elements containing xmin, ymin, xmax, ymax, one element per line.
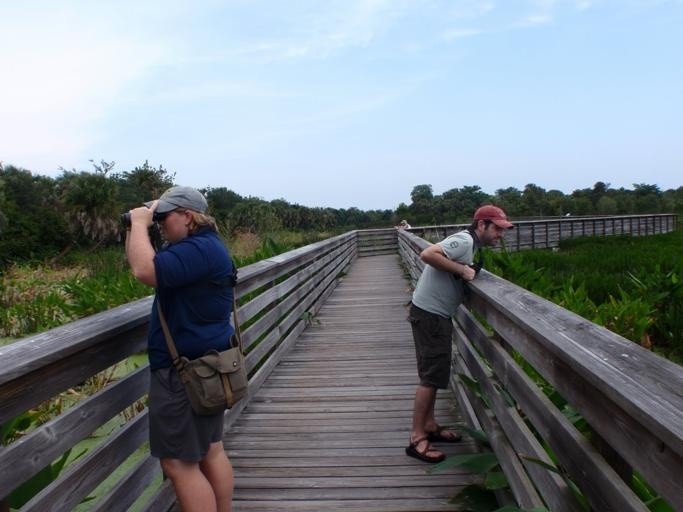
<box><xmin>426</xmin><ymin>425</ymin><xmax>462</xmax><ymax>442</ymax></box>
<box><xmin>405</xmin><ymin>435</ymin><xmax>445</xmax><ymax>463</ymax></box>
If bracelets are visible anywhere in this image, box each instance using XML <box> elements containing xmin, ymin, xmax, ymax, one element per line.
<box><xmin>463</xmin><ymin>264</ymin><xmax>468</xmax><ymax>274</ymax></box>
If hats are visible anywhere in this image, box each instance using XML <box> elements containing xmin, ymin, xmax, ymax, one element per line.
<box><xmin>474</xmin><ymin>206</ymin><xmax>514</xmax><ymax>230</ymax></box>
<box><xmin>143</xmin><ymin>187</ymin><xmax>208</xmax><ymax>213</ymax></box>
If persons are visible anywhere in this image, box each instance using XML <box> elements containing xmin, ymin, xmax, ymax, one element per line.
<box><xmin>400</xmin><ymin>219</ymin><xmax>414</xmax><ymax>234</ymax></box>
<box><xmin>120</xmin><ymin>186</ymin><xmax>237</xmax><ymax>512</ymax></box>
<box><xmin>405</xmin><ymin>204</ymin><xmax>515</xmax><ymax>463</ymax></box>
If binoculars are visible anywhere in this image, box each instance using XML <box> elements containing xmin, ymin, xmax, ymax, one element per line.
<box><xmin>120</xmin><ymin>211</ymin><xmax>165</xmax><ymax>225</ymax></box>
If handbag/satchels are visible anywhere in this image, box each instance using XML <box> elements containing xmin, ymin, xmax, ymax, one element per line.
<box><xmin>182</xmin><ymin>347</ymin><xmax>247</xmax><ymax>415</ymax></box>
